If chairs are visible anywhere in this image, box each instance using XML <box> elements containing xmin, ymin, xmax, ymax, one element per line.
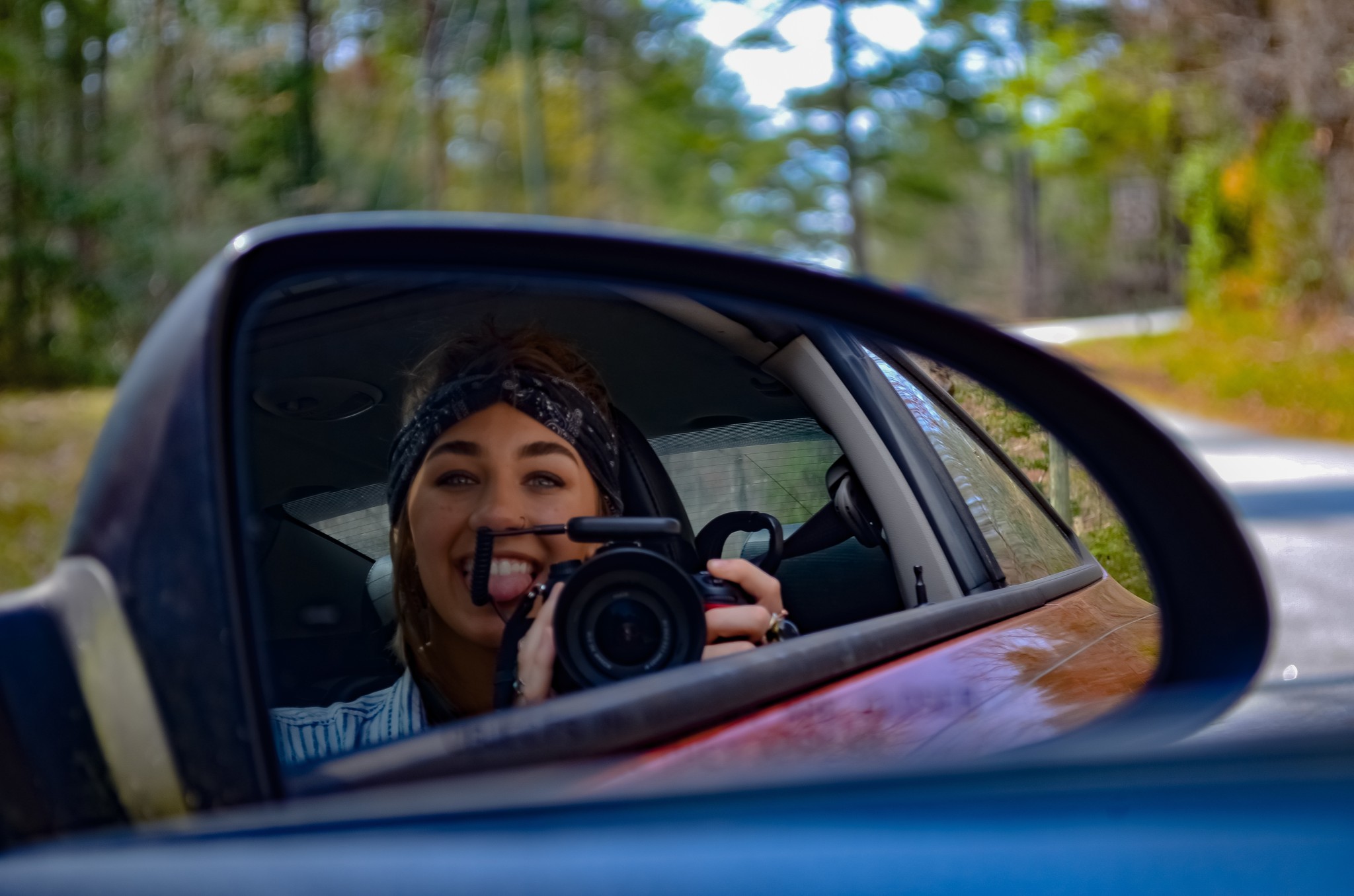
<box><xmin>738</xmin><ymin>522</ymin><xmax>907</xmax><ymax>637</ymax></box>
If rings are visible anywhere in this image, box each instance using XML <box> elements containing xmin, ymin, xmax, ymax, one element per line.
<box><xmin>513</xmin><ymin>677</ymin><xmax>526</xmax><ymax>695</ymax></box>
<box><xmin>755</xmin><ymin>612</ymin><xmax>799</xmax><ymax>647</ymax></box>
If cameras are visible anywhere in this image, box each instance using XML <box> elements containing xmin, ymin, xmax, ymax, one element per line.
<box><xmin>553</xmin><ymin>517</ymin><xmax>757</xmax><ymax>692</ymax></box>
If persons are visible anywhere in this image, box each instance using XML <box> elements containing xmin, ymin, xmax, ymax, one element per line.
<box><xmin>271</xmin><ymin>325</ymin><xmax>798</xmax><ymax>777</ymax></box>
